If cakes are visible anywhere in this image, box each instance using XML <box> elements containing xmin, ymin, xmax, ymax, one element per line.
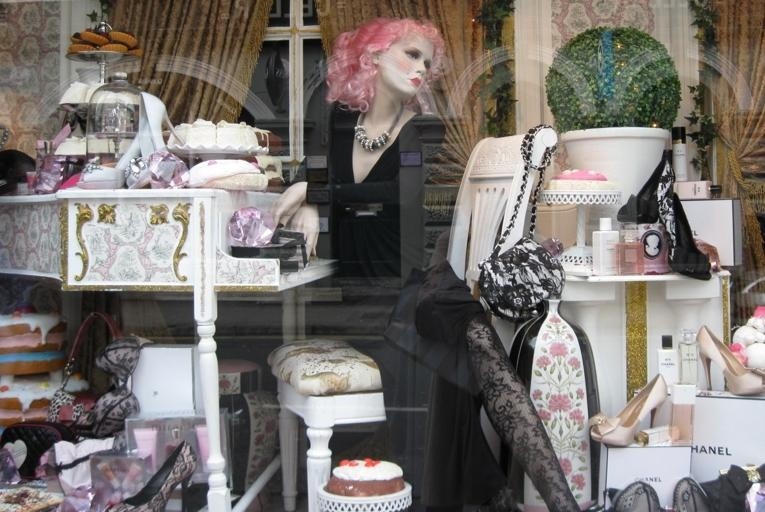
<box><xmin>0</xmin><ymin>304</ymin><xmax>96</xmax><ymax>428</ymax></box>
<box><xmin>65</xmin><ymin>28</ymin><xmax>145</xmax><ymax>59</ymax></box>
<box><xmin>167</xmin><ymin>117</ymin><xmax>270</xmax><ymax>190</ymax></box>
<box><xmin>546</xmin><ymin>168</ymin><xmax>612</xmax><ymax>191</ymax></box>
<box><xmin>0</xmin><ymin>486</ymin><xmax>63</xmax><ymax>512</ymax></box>
<box><xmin>324</xmin><ymin>457</ymin><xmax>405</xmax><ymax>498</ymax></box>
<box><xmin>54</xmin><ymin>81</ymin><xmax>140</xmax><ymax>157</ymax></box>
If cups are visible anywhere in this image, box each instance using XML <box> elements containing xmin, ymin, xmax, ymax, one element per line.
<box><xmin>638</xmin><ymin>224</ymin><xmax>672</xmax><ymax>274</ymax></box>
<box><xmin>669</xmin><ymin>382</ymin><xmax>695</xmax><ymax>446</ymax></box>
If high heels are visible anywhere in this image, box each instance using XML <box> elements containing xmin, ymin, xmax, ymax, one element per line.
<box><xmin>104</xmin><ymin>439</ymin><xmax>198</xmax><ymax>512</ymax></box>
<box><xmin>696</xmin><ymin>324</ymin><xmax>764</xmax><ymax>396</ymax></box>
<box><xmin>590</xmin><ymin>372</ymin><xmax>669</xmax><ymax>447</ymax></box>
<box><xmin>124</xmin><ymin>91</ymin><xmax>185</xmax><ymax>188</ymax></box>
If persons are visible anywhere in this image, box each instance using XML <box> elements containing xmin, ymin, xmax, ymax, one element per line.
<box><xmin>266</xmin><ymin>17</ymin><xmax>581</xmax><ymax>512</ymax></box>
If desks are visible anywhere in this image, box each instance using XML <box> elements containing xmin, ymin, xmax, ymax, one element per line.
<box><xmin>478</xmin><ymin>197</ymin><xmax>765</xmax><ymax>512</ymax></box>
<box><xmin>2</xmin><ymin>190</ymin><xmax>339</xmax><ymax>511</ymax></box>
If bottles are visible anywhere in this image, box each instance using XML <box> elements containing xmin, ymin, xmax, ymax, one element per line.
<box><xmin>658</xmin><ymin>335</ymin><xmax>681</xmax><ymax>393</ymax></box>
<box><xmin>631</xmin><ymin>424</ymin><xmax>680</xmax><ymax>446</ymax></box>
<box><xmin>672</xmin><ymin>127</ymin><xmax>691</xmax><ymax>182</ymax></box>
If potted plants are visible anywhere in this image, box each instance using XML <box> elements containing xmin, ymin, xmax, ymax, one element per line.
<box><xmin>544</xmin><ymin>26</ymin><xmax>682</xmax><ymax>196</ymax></box>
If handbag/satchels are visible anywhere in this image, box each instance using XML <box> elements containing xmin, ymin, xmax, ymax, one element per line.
<box><xmin>475</xmin><ymin>231</ymin><xmax>567</xmax><ymax>322</ymax></box>
<box><xmin>46</xmin><ymin>310</ymin><xmax>121</xmax><ymax>442</ymax></box>
<box><xmin>0</xmin><ymin>420</ymin><xmax>75</xmax><ymax>480</ymax></box>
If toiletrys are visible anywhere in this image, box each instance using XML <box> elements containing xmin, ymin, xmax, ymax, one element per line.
<box><xmin>672</xmin><ymin>127</ymin><xmax>687</xmax><ymax>181</ymax></box>
<box><xmin>658</xmin><ymin>336</ymin><xmax>679</xmax><ymax>387</ymax></box>
<box><xmin>638</xmin><ymin>426</ymin><xmax>681</xmax><ymax>447</ymax></box>
<box><xmin>639</xmin><ymin>212</ymin><xmax>671</xmax><ymax>275</ymax></box>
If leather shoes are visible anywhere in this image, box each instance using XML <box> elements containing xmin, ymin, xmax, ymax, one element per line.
<box><xmin>617</xmin><ymin>157</ymin><xmax>676</xmax><ymax>225</ymax></box>
<box><xmin>76</xmin><ymin>132</ymin><xmax>141</xmax><ymax>191</ymax></box>
<box><xmin>657</xmin><ymin>191</ymin><xmax>713</xmax><ymax>281</ymax></box>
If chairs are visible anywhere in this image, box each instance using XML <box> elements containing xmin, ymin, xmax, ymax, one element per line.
<box><xmin>264</xmin><ymin>127</ymin><xmax>556</xmax><ymax>512</ymax></box>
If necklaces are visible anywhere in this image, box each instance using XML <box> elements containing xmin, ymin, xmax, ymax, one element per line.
<box><xmin>353</xmin><ymin>102</ymin><xmax>404</xmax><ymax>153</ymax></box>
